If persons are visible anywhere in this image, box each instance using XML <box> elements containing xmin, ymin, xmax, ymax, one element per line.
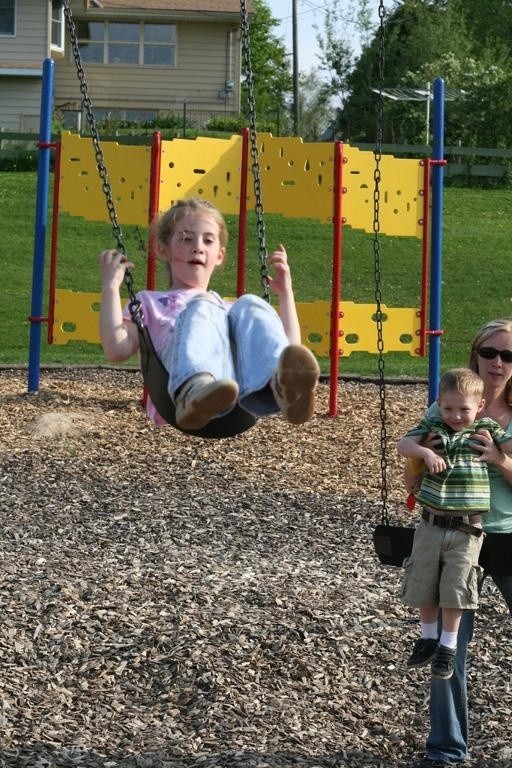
<box><xmin>96</xmin><ymin>196</ymin><xmax>320</xmax><ymax>439</ymax></box>
<box><xmin>401</xmin><ymin>316</ymin><xmax>512</xmax><ymax>768</ymax></box>
<box><xmin>395</xmin><ymin>366</ymin><xmax>511</xmax><ymax>680</ymax></box>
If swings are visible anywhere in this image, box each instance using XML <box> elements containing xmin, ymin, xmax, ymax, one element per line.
<box><xmin>62</xmin><ymin>0</ymin><xmax>268</xmax><ymax>439</ymax></box>
<box><xmin>376</xmin><ymin>1</ymin><xmax>417</xmax><ymax>566</ymax></box>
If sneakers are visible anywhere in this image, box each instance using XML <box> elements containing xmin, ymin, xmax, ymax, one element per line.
<box><xmin>272</xmin><ymin>343</ymin><xmax>320</xmax><ymax>425</ymax></box>
<box><xmin>406</xmin><ymin>638</ymin><xmax>457</xmax><ymax>680</ymax></box>
<box><xmin>174</xmin><ymin>375</ymin><xmax>239</xmax><ymax>431</ymax></box>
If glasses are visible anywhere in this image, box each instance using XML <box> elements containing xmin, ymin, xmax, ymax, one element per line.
<box><xmin>476</xmin><ymin>346</ymin><xmax>511</xmax><ymax>362</ymax></box>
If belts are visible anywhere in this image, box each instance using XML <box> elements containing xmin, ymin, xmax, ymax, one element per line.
<box><xmin>422</xmin><ymin>508</ymin><xmax>483</xmax><ymax>538</ymax></box>
